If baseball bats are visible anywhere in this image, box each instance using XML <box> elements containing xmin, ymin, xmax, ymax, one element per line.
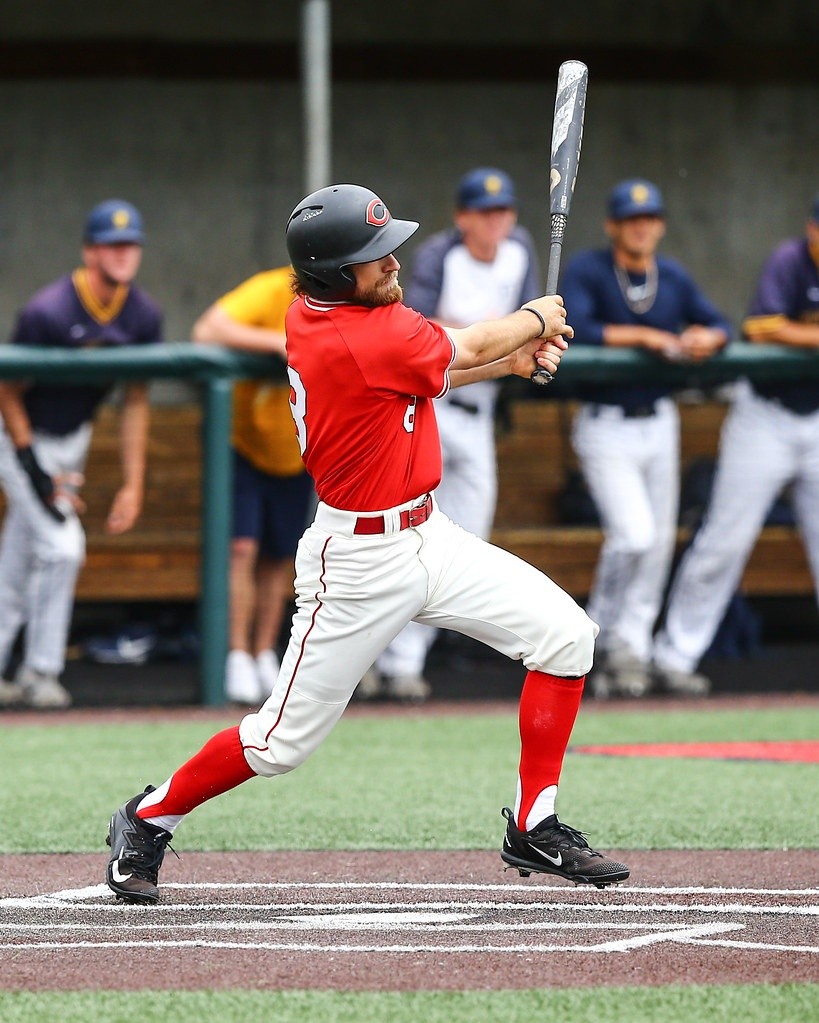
<box><xmin>530</xmin><ymin>58</ymin><xmax>593</xmax><ymax>388</ymax></box>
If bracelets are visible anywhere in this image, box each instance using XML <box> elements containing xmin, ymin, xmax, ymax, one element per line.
<box><xmin>516</xmin><ymin>306</ymin><xmax>545</xmax><ymax>338</ymax></box>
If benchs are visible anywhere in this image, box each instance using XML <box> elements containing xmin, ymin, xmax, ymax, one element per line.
<box><xmin>76</xmin><ymin>398</ymin><xmax>814</xmax><ymax>598</ymax></box>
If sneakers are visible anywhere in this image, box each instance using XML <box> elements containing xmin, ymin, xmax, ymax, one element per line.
<box><xmin>500</xmin><ymin>807</ymin><xmax>630</xmax><ymax>889</ymax></box>
<box><xmin>106</xmin><ymin>785</ymin><xmax>180</xmax><ymax>902</ymax></box>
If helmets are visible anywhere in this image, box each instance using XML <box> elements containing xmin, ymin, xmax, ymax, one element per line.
<box><xmin>286</xmin><ymin>183</ymin><xmax>421</xmax><ymax>301</ymax></box>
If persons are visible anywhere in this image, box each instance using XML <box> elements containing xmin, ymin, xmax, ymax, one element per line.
<box><xmin>648</xmin><ymin>200</ymin><xmax>818</xmax><ymax>698</ymax></box>
<box><xmin>189</xmin><ymin>263</ymin><xmax>315</xmax><ymax>707</ymax></box>
<box><xmin>407</xmin><ymin>166</ymin><xmax>545</xmax><ymax>543</ymax></box>
<box><xmin>553</xmin><ymin>175</ymin><xmax>741</xmax><ymax>702</ymax></box>
<box><xmin>0</xmin><ymin>197</ymin><xmax>163</xmax><ymax>711</ymax></box>
<box><xmin>105</xmin><ymin>60</ymin><xmax>632</xmax><ymax>904</ymax></box>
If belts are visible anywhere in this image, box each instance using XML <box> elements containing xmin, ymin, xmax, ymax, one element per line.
<box><xmin>354</xmin><ymin>497</ymin><xmax>433</xmax><ymax>534</ymax></box>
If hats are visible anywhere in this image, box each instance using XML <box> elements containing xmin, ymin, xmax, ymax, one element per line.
<box><xmin>454</xmin><ymin>166</ymin><xmax>519</xmax><ymax>210</ymax></box>
<box><xmin>82</xmin><ymin>199</ymin><xmax>146</xmax><ymax>249</ymax></box>
<box><xmin>606</xmin><ymin>179</ymin><xmax>665</xmax><ymax>219</ymax></box>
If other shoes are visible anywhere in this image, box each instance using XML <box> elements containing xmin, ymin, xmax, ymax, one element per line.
<box><xmin>224</xmin><ymin>650</ymin><xmax>263</xmax><ymax>705</ymax></box>
<box><xmin>354</xmin><ymin>664</ymin><xmax>384</xmax><ymax>701</ymax></box>
<box><xmin>590</xmin><ymin>644</ymin><xmax>652</xmax><ymax>699</ymax></box>
<box><xmin>258</xmin><ymin>648</ymin><xmax>280</xmax><ymax>702</ymax></box>
<box><xmin>0</xmin><ymin>668</ymin><xmax>72</xmax><ymax>710</ymax></box>
<box><xmin>651</xmin><ymin>668</ymin><xmax>710</xmax><ymax>698</ymax></box>
<box><xmin>384</xmin><ymin>674</ymin><xmax>433</xmax><ymax>703</ymax></box>
